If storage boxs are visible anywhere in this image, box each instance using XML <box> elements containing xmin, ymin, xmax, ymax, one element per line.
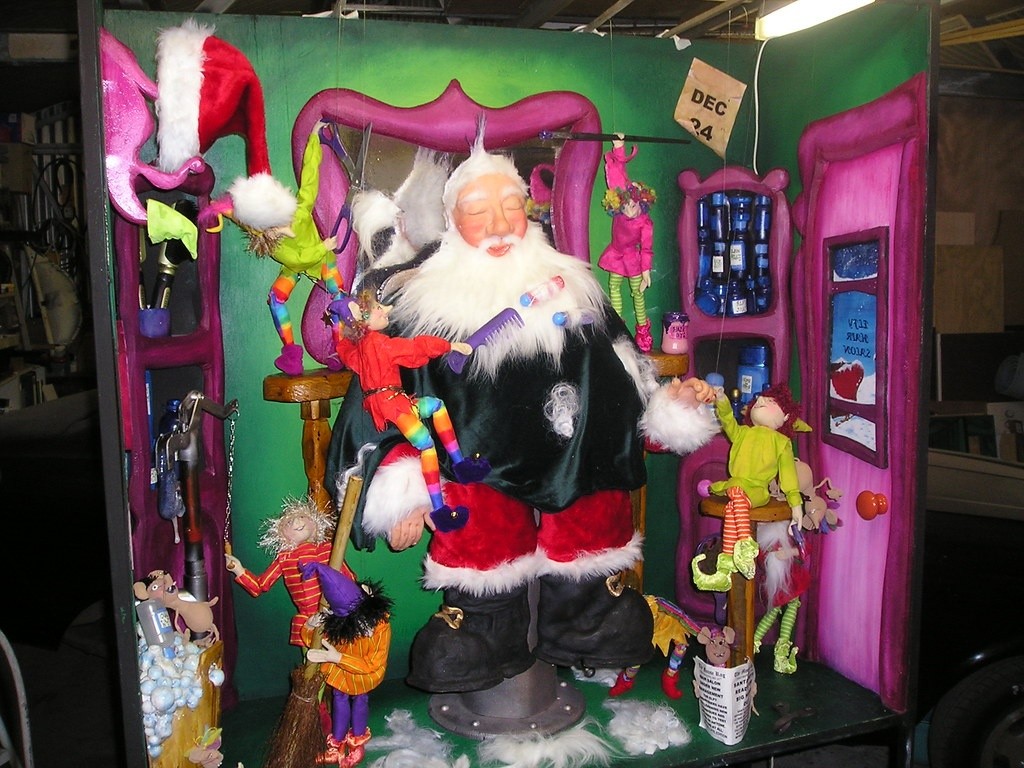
<box><xmin>0</xmin><ymin>143</ymin><xmax>34</xmax><ymax>192</ymax></box>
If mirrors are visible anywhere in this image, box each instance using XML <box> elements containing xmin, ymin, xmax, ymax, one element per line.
<box><xmin>287</xmin><ymin>78</ymin><xmax>602</xmax><ymax>361</ymax></box>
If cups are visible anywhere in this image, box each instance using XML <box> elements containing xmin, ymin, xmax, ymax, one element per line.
<box><xmin>139</xmin><ymin>308</ymin><xmax>171</xmax><ymax>338</ymax></box>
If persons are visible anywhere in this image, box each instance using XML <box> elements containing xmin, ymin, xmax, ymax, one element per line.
<box><xmin>225</xmin><ymin>494</ymin><xmax>358</xmax><ymax>735</ymax></box>
<box><xmin>299</xmin><ymin>562</ymin><xmax>391</xmax><ymax>768</ymax></box>
<box><xmin>354</xmin><ymin>144</ymin><xmax>451</xmax><ymax>296</ymax></box>
<box><xmin>609</xmin><ymin>593</ymin><xmax>701</xmax><ymax>698</ymax></box>
<box><xmin>325</xmin><ymin>292</ymin><xmax>489</xmax><ymax>533</ymax></box>
<box><xmin>597</xmin><ymin>132</ymin><xmax>657</xmax><ymax>352</ymax></box>
<box><xmin>331</xmin><ymin>118</ymin><xmax>718</xmax><ymax>694</ymax></box>
<box><xmin>754</xmin><ymin>519</ymin><xmax>811</xmax><ymax>674</ymax></box>
<box><xmin>229</xmin><ymin>118</ymin><xmax>345</xmax><ymax>375</ymax></box>
<box><xmin>691</xmin><ymin>383</ymin><xmax>803</xmax><ymax>591</ymax></box>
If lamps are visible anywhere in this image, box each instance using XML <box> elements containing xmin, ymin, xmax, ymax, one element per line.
<box><xmin>754</xmin><ymin>0</ymin><xmax>872</xmax><ymax>41</ymax></box>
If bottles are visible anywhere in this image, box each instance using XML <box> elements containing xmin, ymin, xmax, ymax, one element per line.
<box><xmin>136</xmin><ymin>597</ymin><xmax>176</xmax><ymax>661</ymax></box>
<box><xmin>519</xmin><ymin>275</ymin><xmax>565</xmax><ymax>308</ymax></box>
<box><xmin>705</xmin><ymin>344</ymin><xmax>769</xmax><ymax>423</ymax></box>
<box><xmin>552</xmin><ymin>310</ymin><xmax>594</xmax><ymax>326</ymax></box>
<box><xmin>158</xmin><ymin>399</ymin><xmax>182</xmax><ymax>480</ymax></box>
<box><xmin>696</xmin><ymin>190</ymin><xmax>771</xmax><ymax>318</ymax></box>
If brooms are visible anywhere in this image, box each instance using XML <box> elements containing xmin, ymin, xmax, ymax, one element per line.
<box><xmin>260</xmin><ymin>475</ymin><xmax>365</xmax><ymax>768</ymax></box>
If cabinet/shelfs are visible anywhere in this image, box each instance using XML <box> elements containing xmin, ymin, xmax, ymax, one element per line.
<box><xmin>677</xmin><ymin>165</ymin><xmax>787</xmax><ymax>616</ymax></box>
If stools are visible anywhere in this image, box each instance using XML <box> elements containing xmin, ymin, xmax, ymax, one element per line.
<box><xmin>697</xmin><ymin>497</ymin><xmax>791</xmax><ymax>667</ymax></box>
